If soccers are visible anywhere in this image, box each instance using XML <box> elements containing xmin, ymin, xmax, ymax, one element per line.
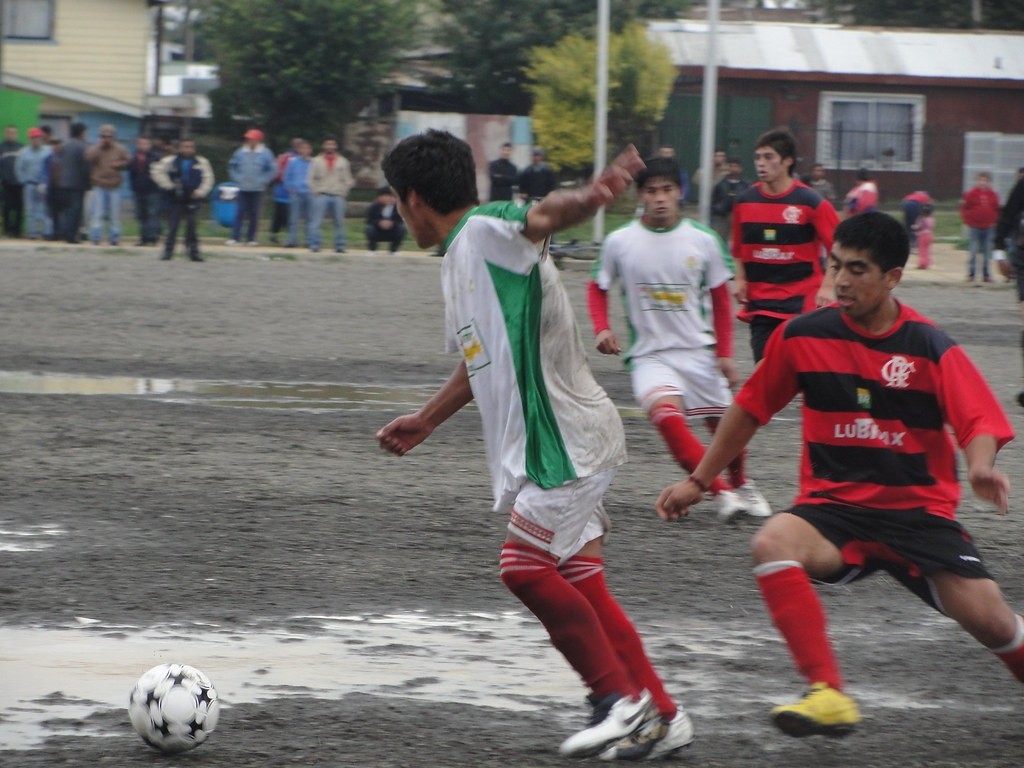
<box><xmin>127</xmin><ymin>662</ymin><xmax>220</xmax><ymax>755</ymax></box>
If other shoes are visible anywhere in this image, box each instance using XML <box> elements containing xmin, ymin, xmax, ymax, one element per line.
<box><xmin>192</xmin><ymin>256</ymin><xmax>203</xmax><ymax>261</ymax></box>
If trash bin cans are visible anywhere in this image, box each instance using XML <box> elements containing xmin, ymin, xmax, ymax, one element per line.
<box><xmin>211</xmin><ymin>181</ymin><xmax>240</xmax><ymax>227</ymax></box>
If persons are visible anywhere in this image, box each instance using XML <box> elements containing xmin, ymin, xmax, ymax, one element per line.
<box><xmin>587</xmin><ymin>154</ymin><xmax>771</xmax><ymax>523</ymax></box>
<box><xmin>657</xmin><ymin>209</ymin><xmax>1024</xmax><ymax>736</ymax></box>
<box><xmin>725</xmin><ymin>126</ymin><xmax>841</xmax><ymax>370</ymax></box>
<box><xmin>0</xmin><ymin>115</ymin><xmax>1023</xmax><ymax>285</ymax></box>
<box><xmin>372</xmin><ymin>127</ymin><xmax>695</xmax><ymax>763</ymax></box>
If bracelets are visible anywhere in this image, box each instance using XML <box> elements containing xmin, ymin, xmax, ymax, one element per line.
<box><xmin>689</xmin><ymin>475</ymin><xmax>706</xmax><ymax>493</ymax></box>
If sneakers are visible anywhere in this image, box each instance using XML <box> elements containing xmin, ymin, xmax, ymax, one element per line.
<box><xmin>715</xmin><ymin>490</ymin><xmax>746</xmax><ymax>523</ymax></box>
<box><xmin>772</xmin><ymin>682</ymin><xmax>859</xmax><ymax>737</ymax></box>
<box><xmin>599</xmin><ymin>701</ymin><xmax>694</xmax><ymax>761</ymax></box>
<box><xmin>560</xmin><ymin>688</ymin><xmax>658</xmax><ymax>758</ymax></box>
<box><xmin>738</xmin><ymin>480</ymin><xmax>772</xmax><ymax>518</ymax></box>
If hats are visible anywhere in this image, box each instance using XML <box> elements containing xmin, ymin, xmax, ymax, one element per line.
<box><xmin>28</xmin><ymin>129</ymin><xmax>43</xmax><ymax>136</ymax></box>
<box><xmin>244</xmin><ymin>129</ymin><xmax>263</xmax><ymax>139</ymax></box>
